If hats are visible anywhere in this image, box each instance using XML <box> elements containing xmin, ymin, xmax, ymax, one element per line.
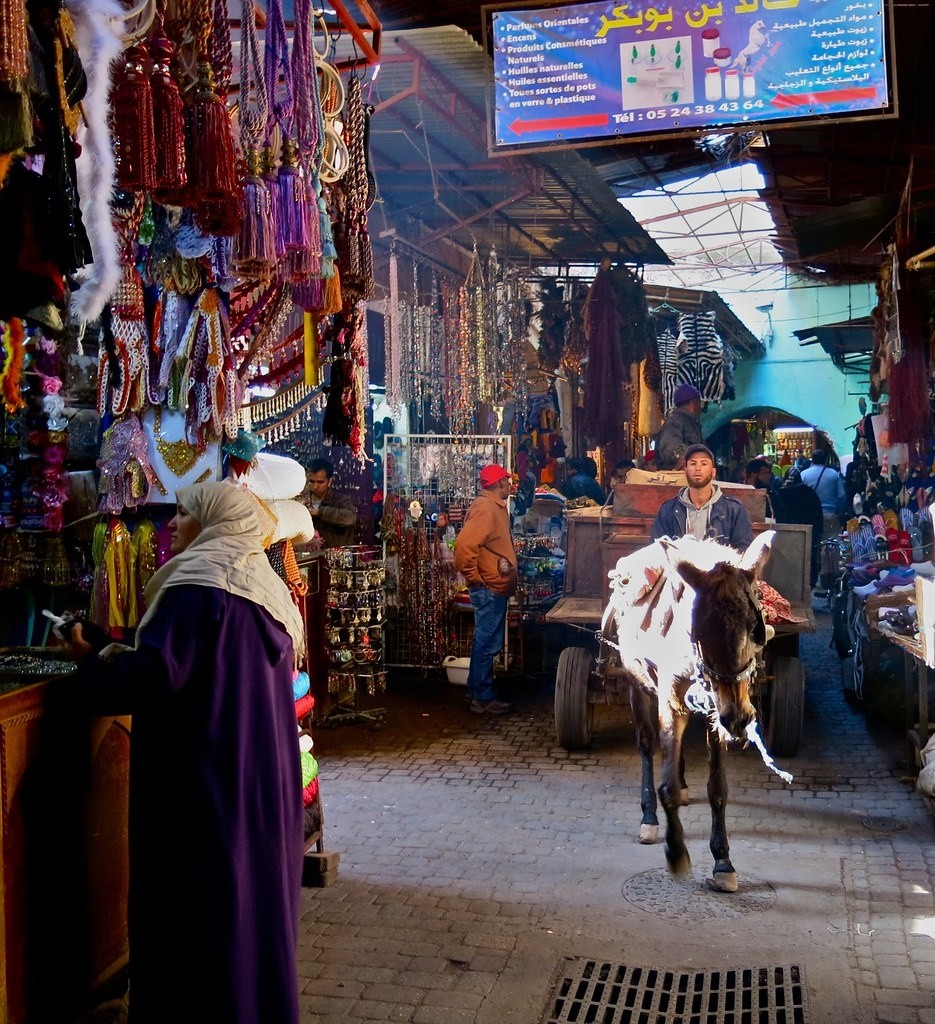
<box><xmin>683</xmin><ymin>444</ymin><xmax>715</xmax><ymax>466</ymax></box>
<box><xmin>674</xmin><ymin>384</ymin><xmax>699</xmax><ymax>404</ymax></box>
<box><xmin>479</xmin><ymin>464</ymin><xmax>511</xmax><ymax>488</ymax></box>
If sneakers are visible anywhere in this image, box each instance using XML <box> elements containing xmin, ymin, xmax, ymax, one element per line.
<box><xmin>470</xmin><ymin>699</ymin><xmax>515</xmax><ymax>714</ymax></box>
<box><xmin>464</xmin><ymin>693</ymin><xmax>471</xmax><ymax>702</ymax></box>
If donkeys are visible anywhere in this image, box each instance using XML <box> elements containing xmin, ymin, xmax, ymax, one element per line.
<box><xmin>609</xmin><ymin>529</ymin><xmax>777</xmax><ymax>893</ymax></box>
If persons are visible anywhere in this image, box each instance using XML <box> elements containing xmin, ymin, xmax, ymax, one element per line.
<box><xmin>454</xmin><ymin>464</ymin><xmax>518</xmax><ymax>712</ymax></box>
<box><xmin>737</xmin><ymin>449</ymin><xmax>856</xmax><ymax>591</ymax></box>
<box><xmin>643</xmin><ymin>450</ymin><xmax>658</xmax><ymax>471</ymax></box>
<box><xmin>655</xmin><ymin>385</ymin><xmax>704</xmax><ymax>471</ymax></box>
<box><xmin>69</xmin><ymin>480</ymin><xmax>304</xmax><ymax>1024</ymax></box>
<box><xmin>649</xmin><ymin>444</ymin><xmax>753</xmax><ymax>553</ymax></box>
<box><xmin>563</xmin><ymin>457</ymin><xmax>636</xmax><ymax>505</ymax></box>
<box><xmin>304</xmin><ymin>458</ymin><xmax>357</xmax><ymax>548</ymax></box>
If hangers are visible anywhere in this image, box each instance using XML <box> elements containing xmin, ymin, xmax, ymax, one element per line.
<box><xmin>603</xmin><ymin>254</ymin><xmax>645</xmax><ymax>285</ymax></box>
<box><xmin>570</xmin><ymin>279</ymin><xmax>592</xmax><ymax>303</ymax></box>
<box><xmin>652</xmin><ymin>297</ymin><xmax>681</xmax><ymax>316</ymax></box>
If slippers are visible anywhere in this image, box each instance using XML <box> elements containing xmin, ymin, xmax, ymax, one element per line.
<box><xmin>849</xmin><ymin>570</ymin><xmax>922</xmax><ymax>645</ymax></box>
<box><xmin>842</xmin><ymin>455</ymin><xmax>935</xmax><ymax>570</ymax></box>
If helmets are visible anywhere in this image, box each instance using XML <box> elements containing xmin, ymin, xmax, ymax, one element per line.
<box><xmin>752</xmin><ymin>454</ymin><xmax>773</xmax><ymax>471</ymax></box>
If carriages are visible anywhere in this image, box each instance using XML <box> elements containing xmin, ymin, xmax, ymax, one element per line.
<box><xmin>537</xmin><ymin>470</ymin><xmax>814</xmax><ymax>758</ymax></box>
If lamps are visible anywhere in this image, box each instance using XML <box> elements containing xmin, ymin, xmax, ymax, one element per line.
<box><xmin>754</xmin><ymin>300</ymin><xmax>774</xmax><ymax>349</ymax></box>
